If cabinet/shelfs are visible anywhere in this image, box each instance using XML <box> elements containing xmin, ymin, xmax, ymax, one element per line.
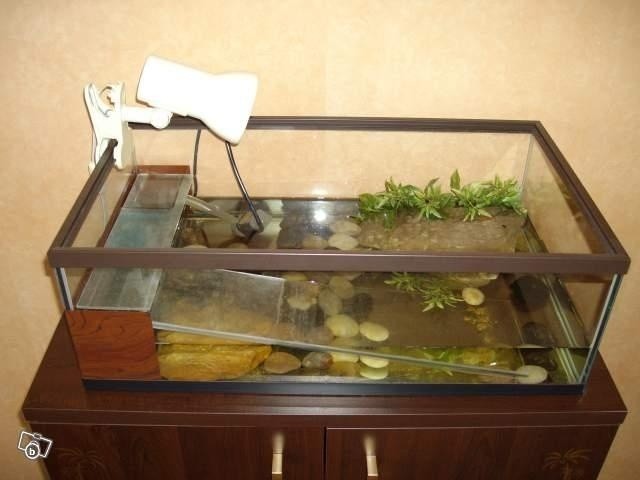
<box><xmin>21</xmin><ymin>195</ymin><xmax>629</xmax><ymax>480</ymax></box>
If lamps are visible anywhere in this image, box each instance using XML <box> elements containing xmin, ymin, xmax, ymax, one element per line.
<box><xmin>84</xmin><ymin>54</ymin><xmax>261</xmax><ymax>171</ymax></box>
